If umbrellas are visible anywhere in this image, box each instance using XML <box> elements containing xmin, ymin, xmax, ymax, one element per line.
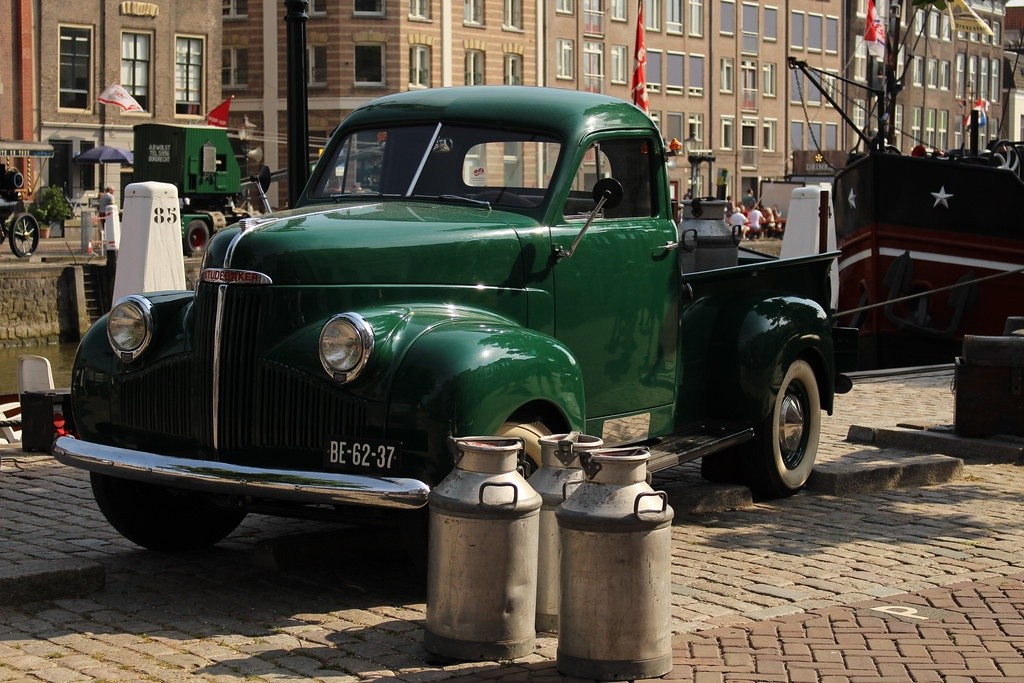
<box><xmin>72</xmin><ymin>146</ymin><xmax>134</xmax><ymax>192</ymax></box>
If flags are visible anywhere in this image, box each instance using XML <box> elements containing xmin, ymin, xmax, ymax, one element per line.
<box><xmin>963</xmin><ymin>101</ymin><xmax>987</xmax><ymax>131</ymax></box>
<box><xmin>631</xmin><ymin>0</ymin><xmax>650</xmax><ymax>153</ymax></box>
<box><xmin>865</xmin><ymin>0</ymin><xmax>885</xmax><ymax>59</ymax></box>
<box><xmin>97</xmin><ymin>82</ymin><xmax>144</xmax><ymax>111</ymax></box>
<box><xmin>208</xmin><ymin>98</ymin><xmax>230</xmax><ymax>127</ymax></box>
<box><xmin>946</xmin><ymin>0</ymin><xmax>996</xmax><ymax>36</ymax></box>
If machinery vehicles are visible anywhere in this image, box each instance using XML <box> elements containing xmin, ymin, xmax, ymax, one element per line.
<box><xmin>118</xmin><ymin>123</ymin><xmax>275</xmax><ymax>257</ymax></box>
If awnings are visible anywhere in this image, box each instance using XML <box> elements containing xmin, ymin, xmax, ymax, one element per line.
<box><xmin>0</xmin><ymin>139</ymin><xmax>55</xmax><ymax>159</ymax></box>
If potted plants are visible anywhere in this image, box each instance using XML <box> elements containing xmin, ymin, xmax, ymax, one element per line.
<box><xmin>39</xmin><ymin>223</ymin><xmax>50</xmax><ymax>239</ymax></box>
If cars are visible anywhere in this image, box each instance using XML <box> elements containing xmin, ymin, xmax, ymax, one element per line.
<box><xmin>0</xmin><ymin>139</ymin><xmax>56</xmax><ymax>257</ymax></box>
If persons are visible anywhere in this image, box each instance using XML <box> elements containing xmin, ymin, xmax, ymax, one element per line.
<box><xmin>725</xmin><ymin>188</ymin><xmax>782</xmax><ymax>242</ymax></box>
<box><xmin>98</xmin><ymin>187</ymin><xmax>115</xmax><ymax>230</ymax></box>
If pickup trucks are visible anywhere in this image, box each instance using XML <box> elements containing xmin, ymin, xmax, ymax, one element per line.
<box><xmin>18</xmin><ymin>86</ymin><xmax>860</xmax><ymax>560</ymax></box>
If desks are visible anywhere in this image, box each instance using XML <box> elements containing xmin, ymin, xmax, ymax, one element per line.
<box><xmin>759</xmin><ymin>217</ymin><xmax>787</xmax><ymax>227</ymax></box>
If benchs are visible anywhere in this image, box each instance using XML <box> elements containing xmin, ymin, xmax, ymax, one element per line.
<box><xmin>745</xmin><ymin>228</ymin><xmax>785</xmax><ymax>239</ymax></box>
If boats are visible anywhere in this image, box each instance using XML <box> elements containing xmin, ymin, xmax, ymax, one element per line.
<box><xmin>786</xmin><ymin>1</ymin><xmax>1023</xmax><ymax>370</ymax></box>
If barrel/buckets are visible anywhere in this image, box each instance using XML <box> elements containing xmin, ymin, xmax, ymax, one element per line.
<box><xmin>525</xmin><ymin>431</ymin><xmax>603</xmax><ymax>633</ymax></box>
<box><xmin>552</xmin><ymin>446</ymin><xmax>675</xmax><ymax>678</ymax></box>
<box><xmin>675</xmin><ymin>195</ymin><xmax>747</xmax><ymax>274</ymax></box>
<box><xmin>424</xmin><ymin>436</ymin><xmax>544</xmax><ymax>660</ymax></box>
